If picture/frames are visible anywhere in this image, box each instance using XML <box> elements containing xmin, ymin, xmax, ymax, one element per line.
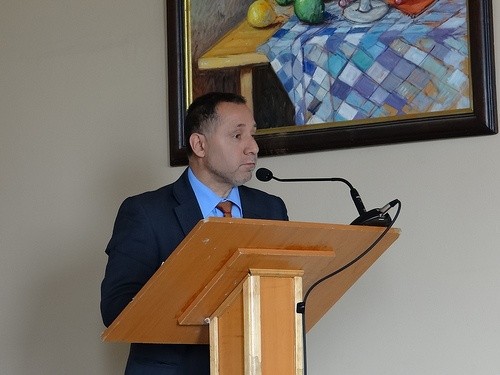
<box><xmin>166</xmin><ymin>0</ymin><xmax>498</xmax><ymax>167</ymax></box>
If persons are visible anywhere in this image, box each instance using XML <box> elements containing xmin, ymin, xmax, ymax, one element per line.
<box><xmin>100</xmin><ymin>92</ymin><xmax>289</xmax><ymax>375</ymax></box>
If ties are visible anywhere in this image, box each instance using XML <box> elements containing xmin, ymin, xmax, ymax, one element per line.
<box><xmin>218</xmin><ymin>201</ymin><xmax>233</xmax><ymax>218</ymax></box>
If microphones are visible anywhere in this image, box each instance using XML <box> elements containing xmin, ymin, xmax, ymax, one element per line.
<box><xmin>256</xmin><ymin>168</ymin><xmax>391</xmax><ymax>228</ymax></box>
